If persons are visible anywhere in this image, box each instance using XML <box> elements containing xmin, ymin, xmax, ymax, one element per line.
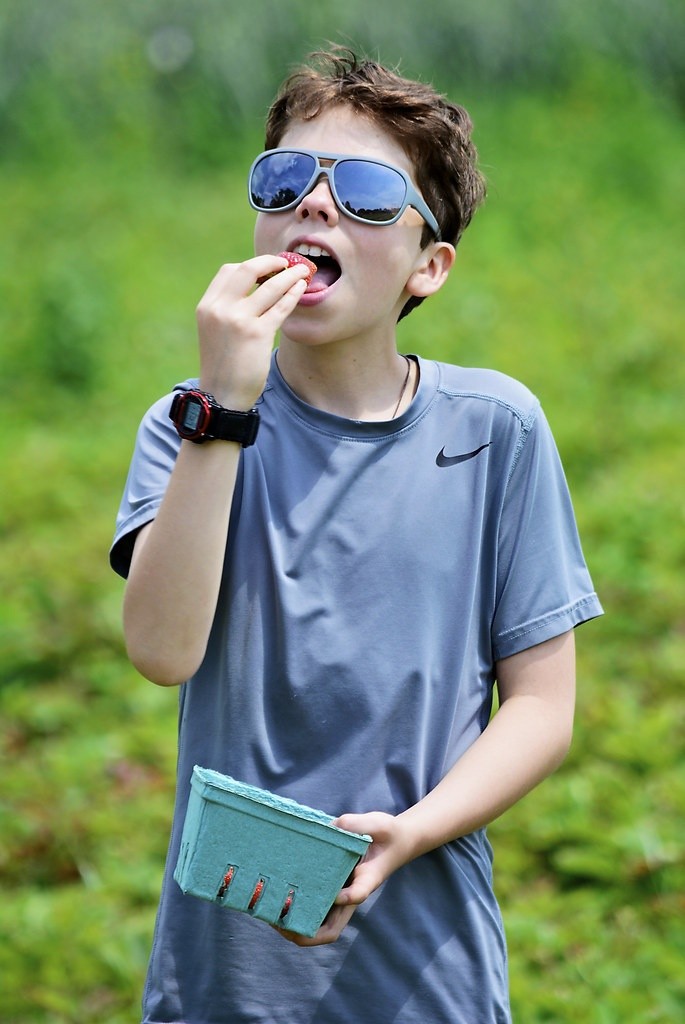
<box><xmin>110</xmin><ymin>41</ymin><xmax>604</xmax><ymax>1024</ymax></box>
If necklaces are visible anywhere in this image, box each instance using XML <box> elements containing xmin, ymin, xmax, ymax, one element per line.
<box><xmin>392</xmin><ymin>353</ymin><xmax>411</xmax><ymax>421</ymax></box>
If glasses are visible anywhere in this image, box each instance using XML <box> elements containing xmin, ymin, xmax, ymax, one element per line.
<box><xmin>247</xmin><ymin>148</ymin><xmax>444</xmax><ymax>243</ymax></box>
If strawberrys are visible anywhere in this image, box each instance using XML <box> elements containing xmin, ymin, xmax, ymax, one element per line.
<box><xmin>261</xmin><ymin>252</ymin><xmax>318</xmax><ymax>287</ymax></box>
<box><xmin>217</xmin><ymin>866</ymin><xmax>295</xmax><ymax>918</ymax></box>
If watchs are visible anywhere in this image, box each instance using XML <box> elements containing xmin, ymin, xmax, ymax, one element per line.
<box><xmin>168</xmin><ymin>388</ymin><xmax>259</xmax><ymax>451</ymax></box>
